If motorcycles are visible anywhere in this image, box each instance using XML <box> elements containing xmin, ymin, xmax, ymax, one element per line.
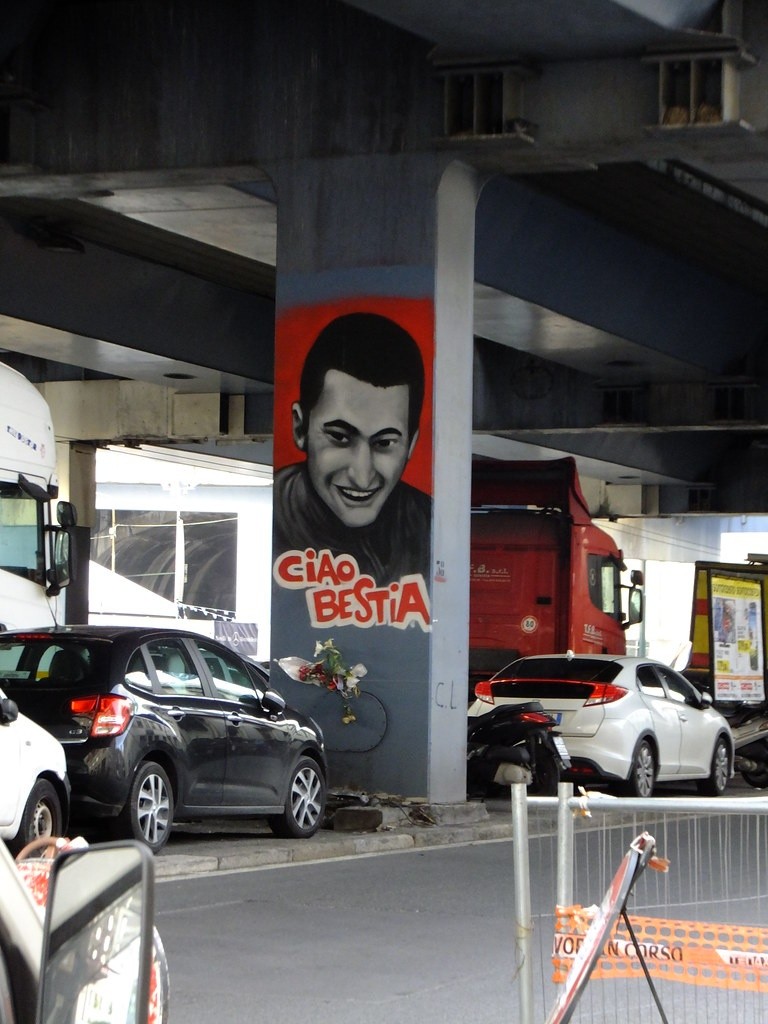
<box><xmin>688</xmin><ymin>677</ymin><xmax>768</xmax><ymax>789</ymax></box>
<box><xmin>466</xmin><ymin>702</ymin><xmax>572</xmax><ymax>797</ymax></box>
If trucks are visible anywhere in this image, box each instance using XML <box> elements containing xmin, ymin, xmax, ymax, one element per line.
<box><xmin>470</xmin><ymin>452</ymin><xmax>645</xmax><ymax>710</ymax></box>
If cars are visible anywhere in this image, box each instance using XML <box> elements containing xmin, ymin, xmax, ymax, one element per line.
<box><xmin>0</xmin><ymin>687</ymin><xmax>72</xmax><ymax>860</ymax></box>
<box><xmin>0</xmin><ymin>620</ymin><xmax>332</xmax><ymax>857</ymax></box>
<box><xmin>476</xmin><ymin>650</ymin><xmax>734</xmax><ymax>800</ymax></box>
